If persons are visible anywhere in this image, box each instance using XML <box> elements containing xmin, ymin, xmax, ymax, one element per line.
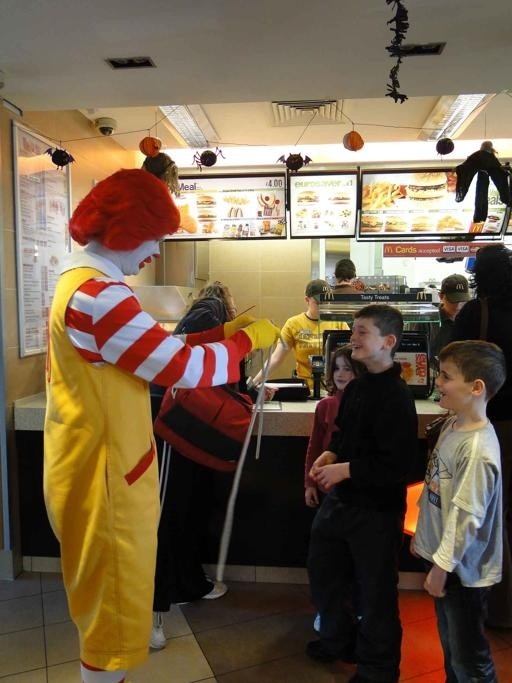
<box><xmin>452</xmin><ymin>244</ymin><xmax>512</xmax><ymax>634</ymax></box>
<box><xmin>43</xmin><ymin>168</ymin><xmax>280</xmax><ymax>678</ymax></box>
<box><xmin>303</xmin><ymin>343</ymin><xmax>367</xmax><ymax>633</ymax></box>
<box><xmin>305</xmin><ymin>303</ymin><xmax>418</xmax><ymax>683</ymax></box>
<box><xmin>409</xmin><ymin>339</ymin><xmax>507</xmax><ymax>682</ymax></box>
<box><xmin>414</xmin><ymin>274</ymin><xmax>471</xmax><ymax>353</ymax></box>
<box><xmin>332</xmin><ymin>257</ymin><xmax>365</xmax><ymax>293</ymax></box>
<box><xmin>144</xmin><ymin>286</ymin><xmax>278</xmax><ymax>650</ymax></box>
<box><xmin>252</xmin><ymin>278</ymin><xmax>350</xmax><ymax>397</ymax></box>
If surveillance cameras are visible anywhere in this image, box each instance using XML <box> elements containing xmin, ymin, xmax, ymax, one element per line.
<box><xmin>94</xmin><ymin>117</ymin><xmax>116</xmax><ymax>137</ymax></box>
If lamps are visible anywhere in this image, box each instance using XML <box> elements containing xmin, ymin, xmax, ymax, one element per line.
<box><xmin>158</xmin><ymin>104</ymin><xmax>221</xmax><ymax>148</ymax></box>
<box><xmin>417</xmin><ymin>94</ymin><xmax>496</xmax><ymax>142</ymax></box>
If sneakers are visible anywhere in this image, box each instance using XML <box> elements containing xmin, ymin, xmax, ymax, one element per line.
<box><xmin>176</xmin><ymin>581</ymin><xmax>227</xmax><ymax>605</ymax></box>
<box><xmin>150</xmin><ymin>611</ymin><xmax>166</xmax><ymax>650</ymax></box>
<box><xmin>306</xmin><ymin>640</ymin><xmax>357</xmax><ymax>664</ymax></box>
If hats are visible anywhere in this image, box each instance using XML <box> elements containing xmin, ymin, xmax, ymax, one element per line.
<box><xmin>306</xmin><ymin>279</ymin><xmax>332</xmax><ymax>302</ymax></box>
<box><xmin>441</xmin><ymin>274</ymin><xmax>470</xmax><ymax>304</ymax></box>
<box><xmin>334</xmin><ymin>259</ymin><xmax>356</xmax><ymax>278</ymax></box>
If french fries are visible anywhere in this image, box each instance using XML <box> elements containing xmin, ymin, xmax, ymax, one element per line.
<box><xmin>362</xmin><ymin>183</ymin><xmax>403</xmax><ymax>210</ymax></box>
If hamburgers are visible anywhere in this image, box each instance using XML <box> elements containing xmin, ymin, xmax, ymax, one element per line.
<box><xmin>360</xmin><ymin>216</ymin><xmax>383</xmax><ymax>231</ymax></box>
<box><xmin>197</xmin><ymin>210</ymin><xmax>216</xmax><ymax>221</ymax></box>
<box><xmin>411</xmin><ymin>216</ymin><xmax>432</xmax><ymax>231</ymax></box>
<box><xmin>385</xmin><ymin>217</ymin><xmax>407</xmax><ymax>231</ymax></box>
<box><xmin>297</xmin><ymin>191</ymin><xmax>319</xmax><ymax>205</ymax></box>
<box><xmin>332</xmin><ymin>193</ymin><xmax>350</xmax><ymax>204</ymax></box>
<box><xmin>408</xmin><ymin>173</ymin><xmax>447</xmax><ymax>198</ymax></box>
<box><xmin>196</xmin><ymin>196</ymin><xmax>217</xmax><ymax>208</ymax></box>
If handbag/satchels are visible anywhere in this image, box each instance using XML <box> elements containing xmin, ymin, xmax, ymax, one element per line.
<box><xmin>153</xmin><ymin>385</ymin><xmax>253</xmax><ymax>473</ymax></box>
